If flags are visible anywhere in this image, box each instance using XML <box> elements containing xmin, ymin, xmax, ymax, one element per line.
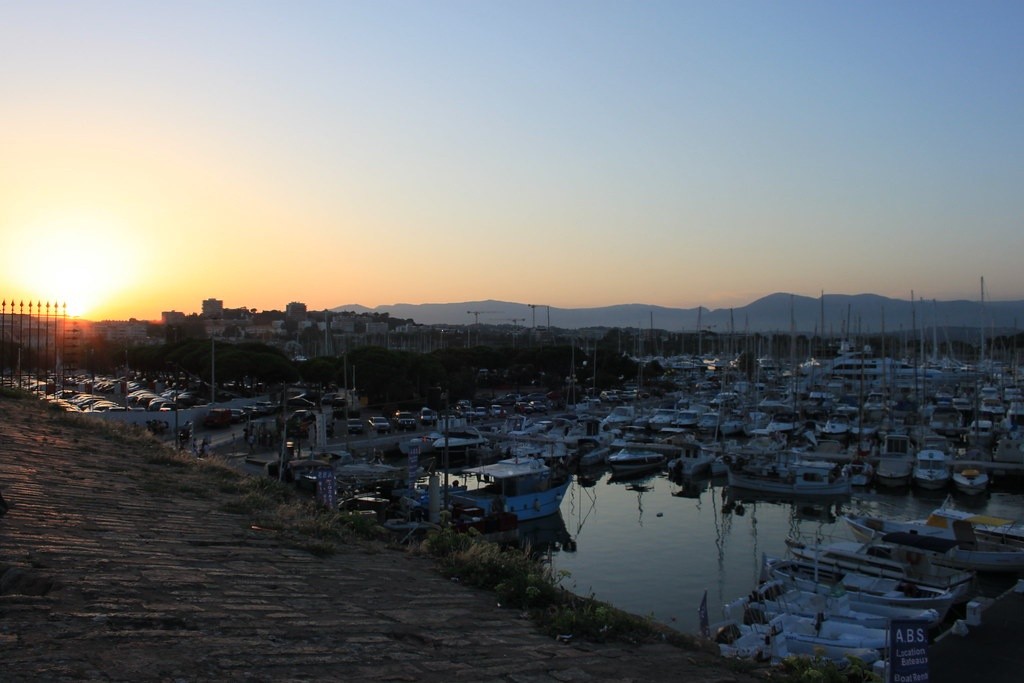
<box><xmin>408</xmin><ymin>446</ymin><xmax>418</xmax><ymax>496</ymax></box>
<box><xmin>700</xmin><ymin>593</ymin><xmax>711</xmax><ymax>640</ymax></box>
<box><xmin>317</xmin><ymin>469</ymin><xmax>336</xmax><ymax>509</ymax></box>
<box><xmin>316</xmin><ymin>414</ymin><xmax>326</xmax><ymax>451</ymax></box>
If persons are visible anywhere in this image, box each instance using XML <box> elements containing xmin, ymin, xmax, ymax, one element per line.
<box><xmin>180</xmin><ymin>413</ymin><xmax>286</xmax><ymax>462</ymax></box>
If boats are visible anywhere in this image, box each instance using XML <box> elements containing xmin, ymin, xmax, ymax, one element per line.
<box><xmin>842</xmin><ymin>506</ymin><xmax>1024</xmax><ymax>575</ymax></box>
<box><xmin>760</xmin><ymin>554</ymin><xmax>957</xmax><ymax>630</ymax></box>
<box><xmin>745</xmin><ymin>580</ymin><xmax>935</xmax><ymax>642</ymax></box>
<box><xmin>292</xmin><ymin>455</ymin><xmax>424</xmax><ymax>491</ymax></box>
<box><xmin>451</xmin><ymin>278</ymin><xmax>1023</xmax><ymax>501</ymax></box>
<box><xmin>435</xmin><ymin>452</ymin><xmax>573</xmax><ymax>520</ymax></box>
<box><xmin>699</xmin><ymin>620</ymin><xmax>880</xmax><ymax>680</ymax></box>
<box><xmin>373</xmin><ymin>494</ymin><xmax>519</xmax><ymax>552</ymax></box>
<box><xmin>786</xmin><ymin>533</ymin><xmax>979</xmax><ymax>607</ymax></box>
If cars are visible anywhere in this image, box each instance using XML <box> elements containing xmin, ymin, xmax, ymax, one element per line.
<box><xmin>0</xmin><ymin>369</ymin><xmax>211</xmax><ymax>417</ymax></box>
<box><xmin>346</xmin><ymin>417</ymin><xmax>363</xmax><ymax>434</ymax></box>
<box><xmin>368</xmin><ymin>416</ymin><xmax>392</xmax><ymax>434</ymax></box>
<box><xmin>242</xmin><ymin>380</ymin><xmax>348</xmax><ymax>432</ymax></box>
<box><xmin>229</xmin><ymin>409</ymin><xmax>246</xmax><ymax>423</ymax></box>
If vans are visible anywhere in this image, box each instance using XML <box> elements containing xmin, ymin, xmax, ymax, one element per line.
<box><xmin>204</xmin><ymin>407</ymin><xmax>232</xmax><ymax>429</ymax></box>
<box><xmin>420</xmin><ymin>407</ymin><xmax>439</xmax><ymax>427</ymax></box>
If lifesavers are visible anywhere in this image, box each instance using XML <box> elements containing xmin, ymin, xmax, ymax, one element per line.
<box><xmin>786</xmin><ymin>474</ymin><xmax>796</xmax><ymax>485</ymax></box>
<box><xmin>906</xmin><ymin>551</ymin><xmax>921</xmax><ymax>565</ymax></box>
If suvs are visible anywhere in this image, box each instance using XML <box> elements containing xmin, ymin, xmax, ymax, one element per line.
<box><xmin>393</xmin><ymin>412</ymin><xmax>416</xmax><ymax>430</ymax></box>
